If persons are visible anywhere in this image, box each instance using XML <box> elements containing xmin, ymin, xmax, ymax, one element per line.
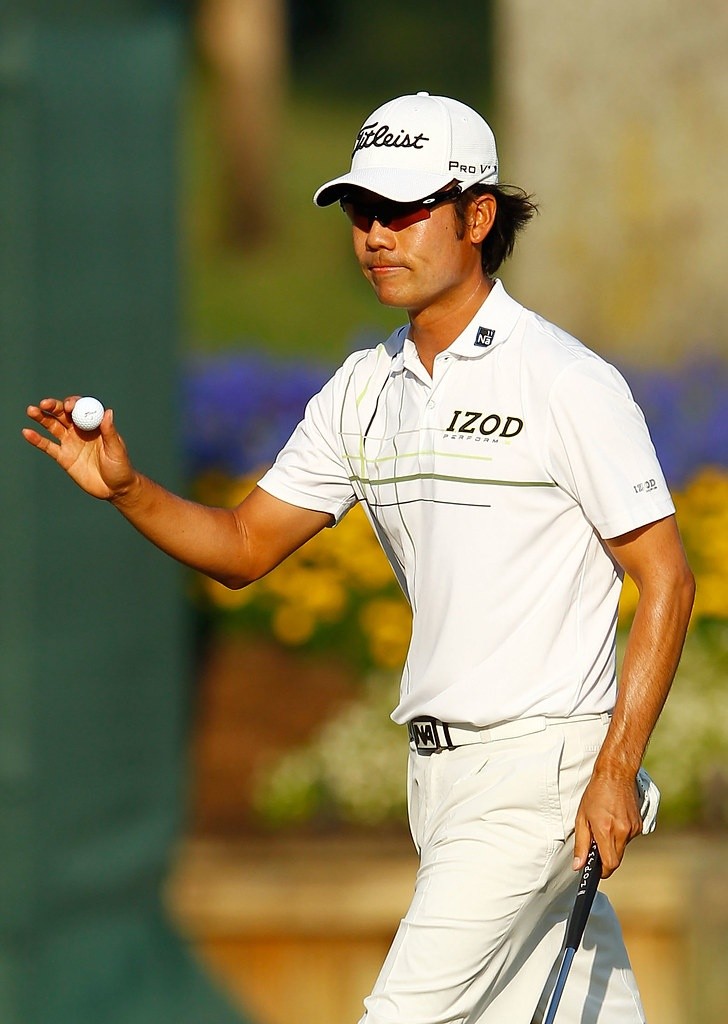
<box><xmin>22</xmin><ymin>89</ymin><xmax>696</xmax><ymax>1024</ymax></box>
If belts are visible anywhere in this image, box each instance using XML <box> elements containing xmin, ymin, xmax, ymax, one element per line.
<box><xmin>407</xmin><ymin>711</ymin><xmax>611</xmax><ymax>750</ymax></box>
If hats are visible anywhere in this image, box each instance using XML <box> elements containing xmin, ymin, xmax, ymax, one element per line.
<box><xmin>313</xmin><ymin>92</ymin><xmax>498</xmax><ymax>209</ymax></box>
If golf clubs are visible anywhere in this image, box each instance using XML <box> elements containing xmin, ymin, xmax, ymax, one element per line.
<box><xmin>529</xmin><ymin>837</ymin><xmax>602</xmax><ymax>1024</ymax></box>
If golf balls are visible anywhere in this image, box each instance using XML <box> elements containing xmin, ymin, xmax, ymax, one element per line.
<box><xmin>71</xmin><ymin>396</ymin><xmax>105</xmax><ymax>431</ymax></box>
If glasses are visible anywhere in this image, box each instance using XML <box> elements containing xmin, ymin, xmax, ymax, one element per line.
<box><xmin>340</xmin><ymin>187</ymin><xmax>457</xmax><ymax>232</ymax></box>
